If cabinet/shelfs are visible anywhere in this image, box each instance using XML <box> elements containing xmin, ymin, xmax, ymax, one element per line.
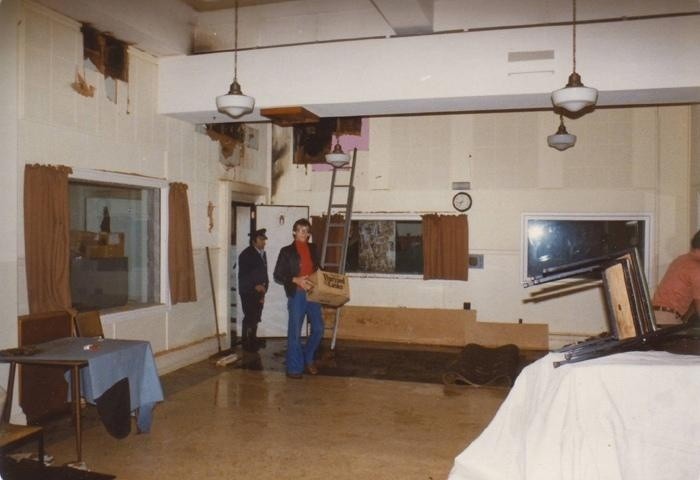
<box><xmin>68</xmin><ymin>256</ymin><xmax>129</xmax><ymax>308</ymax></box>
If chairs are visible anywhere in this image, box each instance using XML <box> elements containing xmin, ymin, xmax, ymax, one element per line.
<box><xmin>75</xmin><ymin>310</ymin><xmax>105</xmax><ymax>341</ymax></box>
<box><xmin>0</xmin><ymin>424</ymin><xmax>44</xmax><ymax>468</ymax></box>
<box><xmin>521</xmin><ymin>246</ymin><xmax>700</xmax><ymax>367</ymax></box>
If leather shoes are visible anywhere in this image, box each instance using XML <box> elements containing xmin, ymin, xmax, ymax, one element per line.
<box><xmin>307</xmin><ymin>363</ymin><xmax>319</xmax><ymax>374</ymax></box>
<box><xmin>287</xmin><ymin>372</ymin><xmax>302</xmax><ymax>378</ymax></box>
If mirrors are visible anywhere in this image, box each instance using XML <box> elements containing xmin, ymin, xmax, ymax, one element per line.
<box><xmin>519</xmin><ymin>212</ymin><xmax>652</xmax><ymax>286</ymax></box>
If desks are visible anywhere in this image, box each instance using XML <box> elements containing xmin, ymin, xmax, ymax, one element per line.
<box><xmin>0</xmin><ymin>339</ymin><xmax>165</xmax><ymax>471</ymax></box>
<box><xmin>445</xmin><ymin>341</ymin><xmax>699</xmax><ymax>480</ymax></box>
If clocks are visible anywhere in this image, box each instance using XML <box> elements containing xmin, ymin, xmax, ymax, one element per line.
<box><xmin>452</xmin><ymin>192</ymin><xmax>473</xmax><ymax>212</ymax></box>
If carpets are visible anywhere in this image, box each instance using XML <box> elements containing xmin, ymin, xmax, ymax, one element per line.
<box><xmin>0</xmin><ymin>456</ymin><xmax>117</xmax><ymax>480</ymax></box>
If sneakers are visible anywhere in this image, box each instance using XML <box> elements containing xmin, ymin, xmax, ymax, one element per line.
<box><xmin>243</xmin><ymin>339</ymin><xmax>256</xmax><ymax>348</ymax></box>
<box><xmin>252</xmin><ymin>335</ymin><xmax>265</xmax><ymax>346</ymax></box>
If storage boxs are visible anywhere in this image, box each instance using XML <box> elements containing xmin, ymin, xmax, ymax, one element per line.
<box><xmin>304</xmin><ymin>269</ymin><xmax>350</xmax><ymax>307</ymax></box>
<box><xmin>68</xmin><ymin>228</ymin><xmax>124</xmax><ymax>259</ymax></box>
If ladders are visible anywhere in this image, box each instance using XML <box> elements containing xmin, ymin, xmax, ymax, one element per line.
<box><xmin>316</xmin><ymin>146</ymin><xmax>357</xmax><ymax>350</ymax></box>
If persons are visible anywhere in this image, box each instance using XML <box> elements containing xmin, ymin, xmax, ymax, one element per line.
<box><xmin>652</xmin><ymin>227</ymin><xmax>700</xmax><ymax>327</ymax></box>
<box><xmin>272</xmin><ymin>216</ymin><xmax>326</xmax><ymax>380</ymax></box>
<box><xmin>235</xmin><ymin>226</ymin><xmax>271</xmax><ymax>353</ymax></box>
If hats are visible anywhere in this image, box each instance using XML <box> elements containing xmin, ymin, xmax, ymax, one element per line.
<box><xmin>246</xmin><ymin>228</ymin><xmax>268</xmax><ymax>241</ymax></box>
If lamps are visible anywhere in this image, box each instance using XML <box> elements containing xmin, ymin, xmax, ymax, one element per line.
<box><xmin>545</xmin><ymin>0</ymin><xmax>599</xmax><ymax>151</ymax></box>
<box><xmin>216</xmin><ymin>0</ymin><xmax>254</xmax><ymax>118</ymax></box>
